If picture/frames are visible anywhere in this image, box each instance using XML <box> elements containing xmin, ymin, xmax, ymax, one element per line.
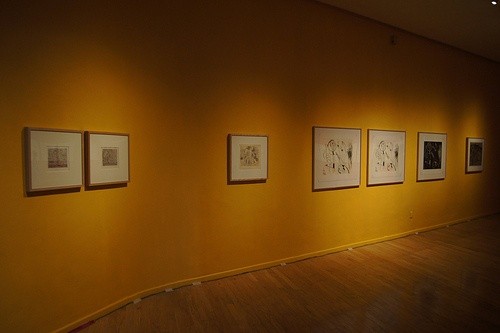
<box><xmin>367</xmin><ymin>129</ymin><xmax>407</xmax><ymax>187</ymax></box>
<box><xmin>85</xmin><ymin>131</ymin><xmax>131</xmax><ymax>187</ymax></box>
<box><xmin>312</xmin><ymin>126</ymin><xmax>362</xmax><ymax>191</ymax></box>
<box><xmin>24</xmin><ymin>126</ymin><xmax>85</xmax><ymax>195</ymax></box>
<box><xmin>465</xmin><ymin>137</ymin><xmax>486</xmax><ymax>174</ymax></box>
<box><xmin>228</xmin><ymin>133</ymin><xmax>269</xmax><ymax>182</ymax></box>
<box><xmin>416</xmin><ymin>132</ymin><xmax>448</xmax><ymax>182</ymax></box>
